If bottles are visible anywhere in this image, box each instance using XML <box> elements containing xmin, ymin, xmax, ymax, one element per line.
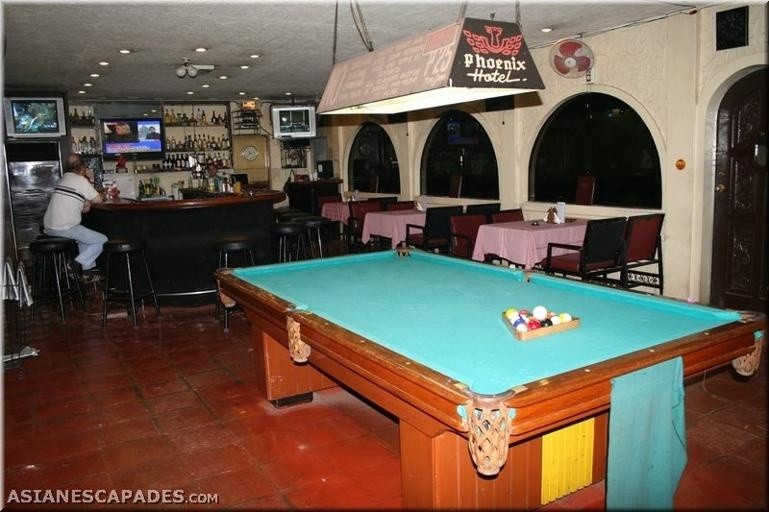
<box><xmin>65</xmin><ymin>105</ymin><xmax>243</xmax><ymax>201</ymax></box>
<box><xmin>290</xmin><ymin>171</ymin><xmax>295</xmax><ymax>182</ymax></box>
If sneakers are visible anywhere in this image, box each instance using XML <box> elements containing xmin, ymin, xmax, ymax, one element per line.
<box><xmin>82</xmin><ymin>271</ymin><xmax>106</xmax><ymax>283</ymax></box>
<box><xmin>62</xmin><ymin>259</ymin><xmax>80</xmax><ymax>280</ymax></box>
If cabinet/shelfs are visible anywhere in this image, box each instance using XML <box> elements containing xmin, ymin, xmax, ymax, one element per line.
<box><xmin>66</xmin><ymin>100</ymin><xmax>234</xmax><ymax>174</ymax></box>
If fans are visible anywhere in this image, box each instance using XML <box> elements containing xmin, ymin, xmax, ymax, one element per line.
<box><xmin>549</xmin><ymin>37</ymin><xmax>596</xmax><ymax>85</ymax></box>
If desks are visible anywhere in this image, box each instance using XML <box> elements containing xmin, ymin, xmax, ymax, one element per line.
<box><xmin>213</xmin><ymin>241</ymin><xmax>767</xmax><ymax>510</ymax></box>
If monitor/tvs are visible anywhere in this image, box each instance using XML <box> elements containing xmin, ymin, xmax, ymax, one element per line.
<box><xmin>3</xmin><ymin>96</ymin><xmax>67</xmax><ymax>140</ymax></box>
<box><xmin>101</xmin><ymin>117</ymin><xmax>166</xmax><ymax>162</ymax></box>
<box><xmin>271</xmin><ymin>105</ymin><xmax>317</xmax><ymax>141</ymax></box>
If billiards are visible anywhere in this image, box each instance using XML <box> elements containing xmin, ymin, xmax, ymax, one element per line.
<box><xmin>505</xmin><ymin>306</ymin><xmax>571</xmax><ymax>333</ymax></box>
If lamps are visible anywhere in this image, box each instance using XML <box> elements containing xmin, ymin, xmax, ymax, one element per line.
<box><xmin>177</xmin><ymin>55</ymin><xmax>198</xmax><ymax>78</ymax></box>
<box><xmin>315</xmin><ymin>1</ymin><xmax>546</xmax><ymax>116</ymax></box>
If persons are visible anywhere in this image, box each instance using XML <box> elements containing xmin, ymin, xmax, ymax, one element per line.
<box><xmin>146</xmin><ymin>127</ymin><xmax>160</xmax><ymax>139</ymax></box>
<box><xmin>203</xmin><ymin>163</ymin><xmax>223</xmax><ymax>190</ymax></box>
<box><xmin>107</xmin><ymin>122</ymin><xmax>138</xmax><ymax>143</ymax></box>
<box><xmin>41</xmin><ymin>151</ymin><xmax>109</xmax><ymax>285</ymax></box>
